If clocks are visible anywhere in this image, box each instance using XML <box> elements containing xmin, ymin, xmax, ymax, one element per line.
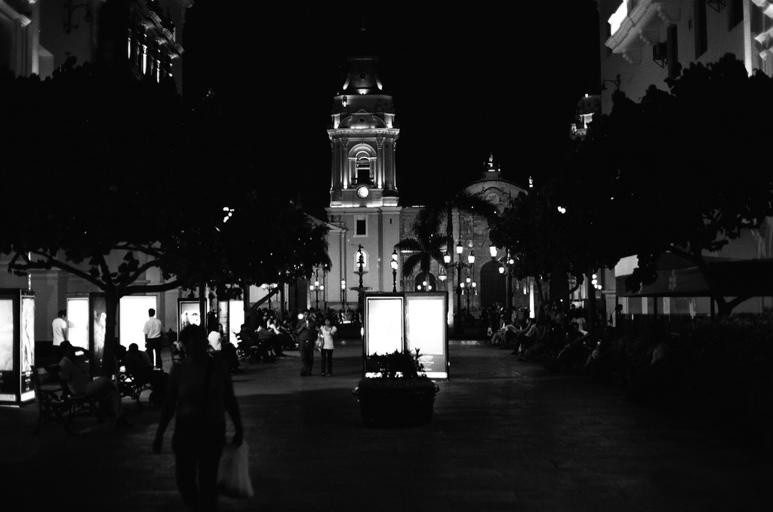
<box><xmin>356</xmin><ymin>186</ymin><xmax>370</xmax><ymax>200</ymax></box>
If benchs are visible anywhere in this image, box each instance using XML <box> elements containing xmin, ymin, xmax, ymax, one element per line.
<box><xmin>24</xmin><ymin>326</ymin><xmax>297</xmax><ymax>437</ymax></box>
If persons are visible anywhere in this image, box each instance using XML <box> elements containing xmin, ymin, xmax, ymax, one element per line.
<box><xmin>152</xmin><ymin>325</ymin><xmax>244</xmax><ymax>512</ymax></box>
<box><xmin>57</xmin><ymin>340</ymin><xmax>122</xmax><ymax>418</ymax></box>
<box><xmin>51</xmin><ymin>311</ymin><xmax>67</xmax><ymax>369</ymax></box>
<box><xmin>124</xmin><ymin>343</ymin><xmax>155</xmax><ymax>383</ymax></box>
<box><xmin>455</xmin><ymin>302</ymin><xmax>624</xmax><ymax>370</ymax></box>
<box><xmin>147</xmin><ymin>309</ymin><xmax>167</xmax><ymax>369</ymax></box>
<box><xmin>242</xmin><ymin>304</ymin><xmax>360</xmax><ymax>379</ymax></box>
<box><xmin>650</xmin><ymin>336</ymin><xmax>670</xmax><ymax>401</ymax></box>
<box><xmin>207</xmin><ymin>324</ymin><xmax>224</xmax><ymax>359</ymax></box>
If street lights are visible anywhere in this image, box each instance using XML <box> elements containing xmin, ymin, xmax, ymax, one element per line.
<box><xmin>459</xmin><ymin>276</ymin><xmax>477</xmax><ymax>314</ymax></box>
<box><xmin>390</xmin><ymin>251</ymin><xmax>400</xmax><ymax>291</ymax></box>
<box><xmin>309</xmin><ymin>279</ymin><xmax>326</xmax><ymax>311</ymax></box>
<box><xmin>489</xmin><ymin>245</ymin><xmax>515</xmax><ymax>308</ymax></box>
<box><xmin>443</xmin><ymin>240</ymin><xmax>475</xmax><ymax>321</ymax></box>
<box><xmin>340</xmin><ymin>278</ymin><xmax>346</xmax><ymax>307</ymax></box>
<box><xmin>262</xmin><ymin>283</ymin><xmax>278</xmax><ymax>308</ymax></box>
<box><xmin>415</xmin><ymin>280</ymin><xmax>432</xmax><ymax>291</ymax></box>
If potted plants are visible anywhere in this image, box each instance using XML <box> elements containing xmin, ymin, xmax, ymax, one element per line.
<box><xmin>351</xmin><ymin>346</ymin><xmax>441</xmax><ymax>428</ymax></box>
